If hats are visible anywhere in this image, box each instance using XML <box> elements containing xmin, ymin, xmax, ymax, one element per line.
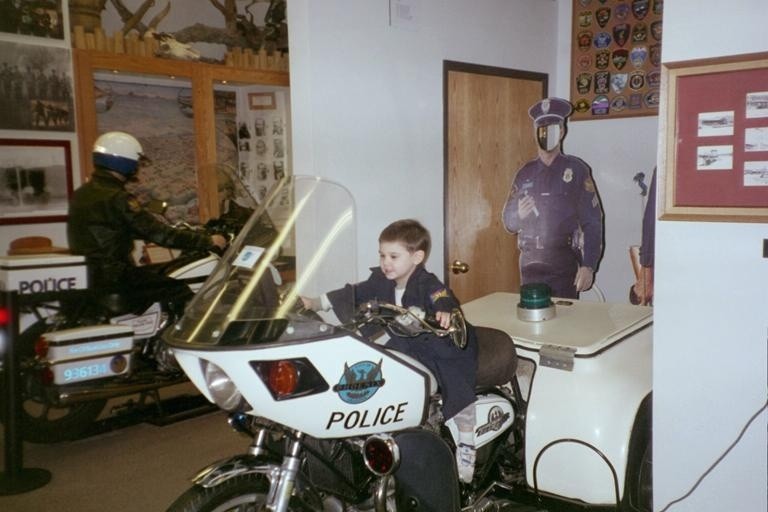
<box><xmin>527</xmin><ymin>97</ymin><xmax>574</xmax><ymax>128</ymax></box>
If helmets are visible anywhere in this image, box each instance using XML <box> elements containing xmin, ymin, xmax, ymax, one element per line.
<box><xmin>92</xmin><ymin>130</ymin><xmax>145</xmax><ymax>183</ymax></box>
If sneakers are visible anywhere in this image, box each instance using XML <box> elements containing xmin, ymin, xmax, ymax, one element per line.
<box><xmin>455</xmin><ymin>439</ymin><xmax>478</xmax><ymax>484</ymax></box>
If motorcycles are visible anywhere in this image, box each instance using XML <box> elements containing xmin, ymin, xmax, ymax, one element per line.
<box><xmin>165</xmin><ymin>174</ymin><xmax>537</xmax><ymax>511</ymax></box>
<box><xmin>0</xmin><ymin>162</ymin><xmax>282</xmax><ymax>444</ymax></box>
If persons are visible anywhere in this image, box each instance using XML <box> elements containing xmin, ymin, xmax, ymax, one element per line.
<box><xmin>67</xmin><ymin>131</ymin><xmax>228</xmax><ymax>379</ymax></box>
<box><xmin>501</xmin><ymin>96</ymin><xmax>605</xmax><ymax>299</ymax></box>
<box><xmin>634</xmin><ymin>165</ymin><xmax>657</xmax><ymax>306</ymax></box>
<box><xmin>278</xmin><ymin>219</ymin><xmax>479</xmax><ymax>485</ymax></box>
